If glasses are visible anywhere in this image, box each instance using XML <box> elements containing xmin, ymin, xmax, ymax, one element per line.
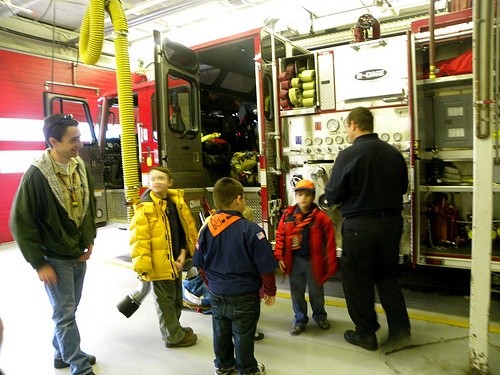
<box><xmin>45</xmin><ymin>114</ymin><xmax>75</xmax><ymax>150</ymax></box>
<box><xmin>240</xmin><ymin>197</ymin><xmax>247</xmax><ymax>200</ymax></box>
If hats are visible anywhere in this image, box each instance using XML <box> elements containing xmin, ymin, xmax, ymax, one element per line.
<box><xmin>294</xmin><ymin>180</ymin><xmax>316</xmax><ymax>192</ymax></box>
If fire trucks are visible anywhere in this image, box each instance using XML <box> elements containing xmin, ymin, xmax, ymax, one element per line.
<box><xmin>41</xmin><ymin>0</ymin><xmax>500</xmax><ymax>274</ymax></box>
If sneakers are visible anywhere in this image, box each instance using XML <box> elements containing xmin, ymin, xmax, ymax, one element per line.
<box><xmin>182</xmin><ymin>327</ymin><xmax>192</xmax><ymax>334</ymax></box>
<box><xmin>255</xmin><ymin>330</ymin><xmax>265</xmax><ymax>341</ymax></box>
<box><xmin>215</xmin><ymin>357</ymin><xmax>236</xmax><ymax>375</ymax></box>
<box><xmin>290</xmin><ymin>324</ymin><xmax>305</xmax><ymax>336</ymax></box>
<box><xmin>319</xmin><ymin>319</ymin><xmax>330</xmax><ymax>330</ymax></box>
<box><xmin>165</xmin><ymin>331</ymin><xmax>197</xmax><ymax>348</ymax></box>
<box><xmin>235</xmin><ymin>362</ymin><xmax>265</xmax><ymax>375</ymax></box>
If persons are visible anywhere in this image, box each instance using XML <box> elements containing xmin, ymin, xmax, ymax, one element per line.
<box><xmin>130</xmin><ymin>167</ymin><xmax>198</xmax><ymax>348</ymax></box>
<box><xmin>274</xmin><ymin>180</ymin><xmax>337</xmax><ymax>335</ymax></box>
<box><xmin>324</xmin><ymin>107</ymin><xmax>412</xmax><ymax>350</ymax></box>
<box><xmin>9</xmin><ymin>113</ymin><xmax>96</xmax><ymax>375</ymax></box>
<box><xmin>192</xmin><ymin>177</ymin><xmax>279</xmax><ymax>375</ymax></box>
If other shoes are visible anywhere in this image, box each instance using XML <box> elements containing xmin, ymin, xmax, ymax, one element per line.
<box><xmin>52</xmin><ymin>352</ymin><xmax>97</xmax><ymax>368</ymax></box>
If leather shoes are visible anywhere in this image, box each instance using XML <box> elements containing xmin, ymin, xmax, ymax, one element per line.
<box><xmin>344</xmin><ymin>329</ymin><xmax>378</xmax><ymax>351</ymax></box>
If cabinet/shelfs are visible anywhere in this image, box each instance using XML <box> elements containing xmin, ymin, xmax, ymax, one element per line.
<box><xmin>407</xmin><ymin>7</ymin><xmax>500</xmax><ymax>269</ymax></box>
